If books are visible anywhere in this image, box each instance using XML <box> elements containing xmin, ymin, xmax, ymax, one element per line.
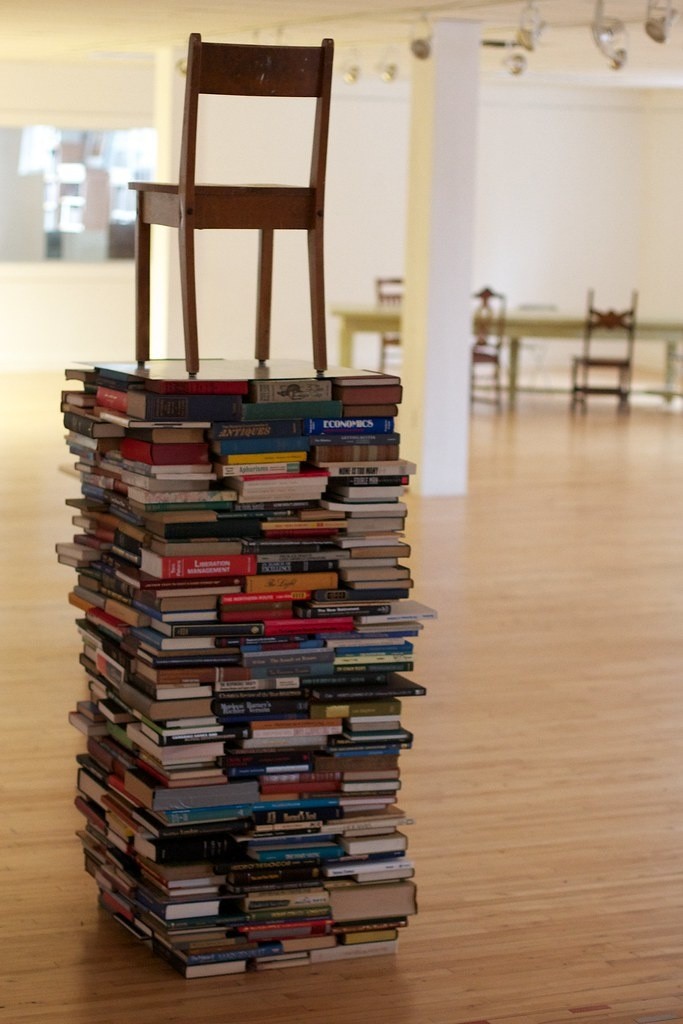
<box><xmin>54</xmin><ymin>358</ymin><xmax>436</xmax><ymax>980</ymax></box>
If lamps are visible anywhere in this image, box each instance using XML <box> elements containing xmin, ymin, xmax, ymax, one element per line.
<box><xmin>581</xmin><ymin>0</ymin><xmax>629</xmax><ymax>72</ymax></box>
<box><xmin>498</xmin><ymin>3</ymin><xmax>546</xmax><ymax>78</ymax></box>
<box><xmin>408</xmin><ymin>12</ymin><xmax>435</xmax><ymax>59</ymax></box>
<box><xmin>643</xmin><ymin>1</ymin><xmax>677</xmax><ymax>44</ymax></box>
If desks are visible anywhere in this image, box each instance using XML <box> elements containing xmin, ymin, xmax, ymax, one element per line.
<box><xmin>329</xmin><ymin>306</ymin><xmax>683</xmax><ymax>404</ymax></box>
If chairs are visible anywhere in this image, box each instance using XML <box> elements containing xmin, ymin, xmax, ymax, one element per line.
<box><xmin>378</xmin><ymin>279</ymin><xmax>403</xmax><ymax>373</ymax></box>
<box><xmin>470</xmin><ymin>290</ymin><xmax>505</xmax><ymax>411</ymax></box>
<box><xmin>129</xmin><ymin>34</ymin><xmax>334</xmax><ymax>379</ymax></box>
<box><xmin>570</xmin><ymin>291</ymin><xmax>638</xmax><ymax>403</ymax></box>
